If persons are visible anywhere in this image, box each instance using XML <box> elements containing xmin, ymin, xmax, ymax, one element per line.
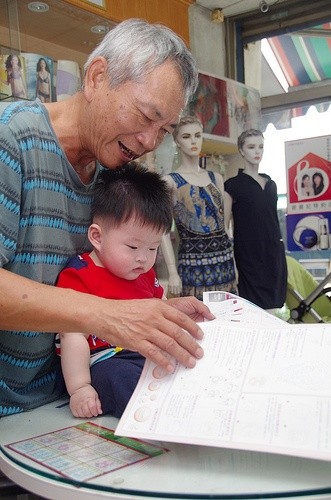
<box><xmin>5</xmin><ymin>54</ymin><xmax>28</xmax><ymax>101</ymax></box>
<box><xmin>35</xmin><ymin>57</ymin><xmax>51</xmax><ymax>103</ymax></box>
<box><xmin>0</xmin><ymin>18</ymin><xmax>216</xmax><ymax>496</ymax></box>
<box><xmin>221</xmin><ymin>129</ymin><xmax>287</xmax><ymax>309</ymax></box>
<box><xmin>53</xmin><ymin>162</ymin><xmax>179</xmax><ymax>425</ymax></box>
<box><xmin>301</xmin><ymin>172</ymin><xmax>324</xmax><ymax>197</ymax></box>
<box><xmin>158</xmin><ymin>114</ymin><xmax>239</xmax><ymax>306</ymax></box>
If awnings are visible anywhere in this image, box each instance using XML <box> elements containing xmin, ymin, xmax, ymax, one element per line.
<box><xmin>261</xmin><ymin>19</ymin><xmax>331</xmax><ymax>132</ymax></box>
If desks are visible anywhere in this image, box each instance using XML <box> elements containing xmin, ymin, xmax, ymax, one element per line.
<box><xmin>0</xmin><ymin>392</ymin><xmax>331</xmax><ymax>500</ymax></box>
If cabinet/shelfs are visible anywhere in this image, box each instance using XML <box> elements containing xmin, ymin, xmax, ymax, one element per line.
<box><xmin>0</xmin><ymin>0</ymin><xmax>262</xmax><ymax>168</ymax></box>
